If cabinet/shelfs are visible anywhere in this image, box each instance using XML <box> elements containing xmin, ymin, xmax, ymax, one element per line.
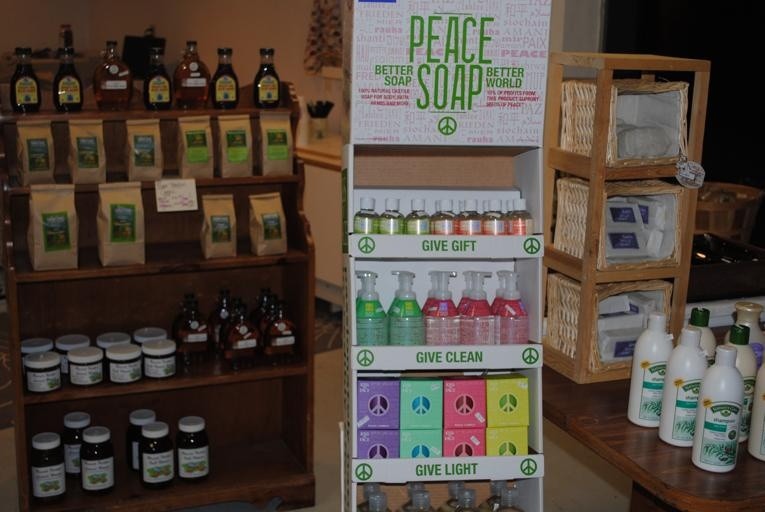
<box><xmin>1</xmin><ymin>76</ymin><xmax>319</xmax><ymax>512</ymax></box>
<box><xmin>340</xmin><ymin>139</ymin><xmax>544</xmax><ymax>511</ymax></box>
<box><xmin>538</xmin><ymin>52</ymin><xmax>712</xmax><ymax>385</ymax></box>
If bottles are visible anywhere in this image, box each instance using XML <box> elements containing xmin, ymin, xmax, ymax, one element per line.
<box><xmin>353</xmin><ymin>195</ymin><xmax>535</xmax><ymax>235</ymax></box>
<box><xmin>31</xmin><ymin>407</ymin><xmax>213</xmax><ymax>499</ymax></box>
<box><xmin>52</xmin><ymin>45</ymin><xmax>84</xmax><ymax>111</ymax></box>
<box><xmin>176</xmin><ymin>288</ymin><xmax>299</xmax><ymax>373</ymax></box>
<box><xmin>91</xmin><ymin>39</ymin><xmax>134</xmax><ymax>112</ymax></box>
<box><xmin>171</xmin><ymin>39</ymin><xmax>213</xmax><ymax>110</ymax></box>
<box><xmin>10</xmin><ymin>45</ymin><xmax>40</xmax><ymax>111</ymax></box>
<box><xmin>623</xmin><ymin>299</ymin><xmax>764</xmax><ymax>474</ymax></box>
<box><xmin>207</xmin><ymin>48</ymin><xmax>240</xmax><ymax>108</ymax></box>
<box><xmin>142</xmin><ymin>37</ymin><xmax>173</xmax><ymax>112</ymax></box>
<box><xmin>253</xmin><ymin>47</ymin><xmax>279</xmax><ymax>106</ymax></box>
<box><xmin>357</xmin><ymin>481</ymin><xmax>527</xmax><ymax>512</ymax></box>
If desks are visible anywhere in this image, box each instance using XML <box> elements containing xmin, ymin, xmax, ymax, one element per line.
<box><xmin>292</xmin><ymin>131</ymin><xmax>343</xmax><ymax>314</ymax></box>
<box><xmin>536</xmin><ymin>325</ymin><xmax>765</xmax><ymax>511</ymax></box>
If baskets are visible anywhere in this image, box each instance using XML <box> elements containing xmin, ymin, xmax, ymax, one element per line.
<box><xmin>551</xmin><ymin>176</ymin><xmax>685</xmax><ymax>273</ymax></box>
<box><xmin>545</xmin><ymin>273</ymin><xmax>677</xmax><ymax>376</ymax></box>
<box><xmin>560</xmin><ymin>75</ymin><xmax>689</xmax><ymax>171</ymax></box>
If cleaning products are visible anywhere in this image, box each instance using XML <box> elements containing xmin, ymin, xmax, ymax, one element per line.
<box><xmin>356</xmin><ymin>270</ymin><xmax>532</xmax><ymax>346</ymax></box>
<box><xmin>626</xmin><ymin>301</ymin><xmax>765</xmax><ymax>474</ymax></box>
